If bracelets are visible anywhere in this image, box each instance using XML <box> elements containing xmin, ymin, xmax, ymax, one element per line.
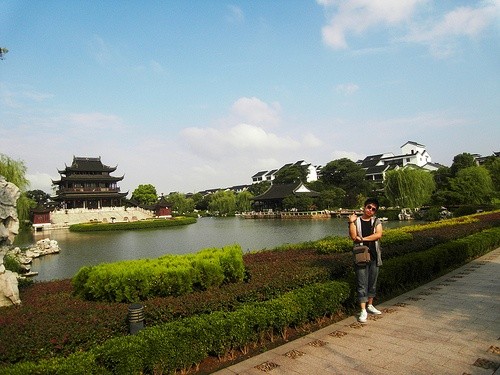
<box><xmin>349</xmin><ymin>221</ymin><xmax>354</xmax><ymax>224</ymax></box>
<box><xmin>360</xmin><ymin>237</ymin><xmax>363</xmax><ymax>242</ymax></box>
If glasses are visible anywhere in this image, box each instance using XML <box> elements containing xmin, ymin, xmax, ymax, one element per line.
<box><xmin>367</xmin><ymin>205</ymin><xmax>376</xmax><ymax>211</ymax></box>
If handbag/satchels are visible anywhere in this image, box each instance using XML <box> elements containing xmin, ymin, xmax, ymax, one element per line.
<box><xmin>353</xmin><ymin>246</ymin><xmax>371</xmax><ymax>264</ymax></box>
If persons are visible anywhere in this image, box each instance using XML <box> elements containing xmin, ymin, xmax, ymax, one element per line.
<box><xmin>348</xmin><ymin>198</ymin><xmax>383</xmax><ymax>322</ymax></box>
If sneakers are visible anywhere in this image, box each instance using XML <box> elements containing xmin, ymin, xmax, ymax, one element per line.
<box><xmin>366</xmin><ymin>306</ymin><xmax>382</xmax><ymax>314</ymax></box>
<box><xmin>358</xmin><ymin>312</ymin><xmax>367</xmax><ymax>322</ymax></box>
